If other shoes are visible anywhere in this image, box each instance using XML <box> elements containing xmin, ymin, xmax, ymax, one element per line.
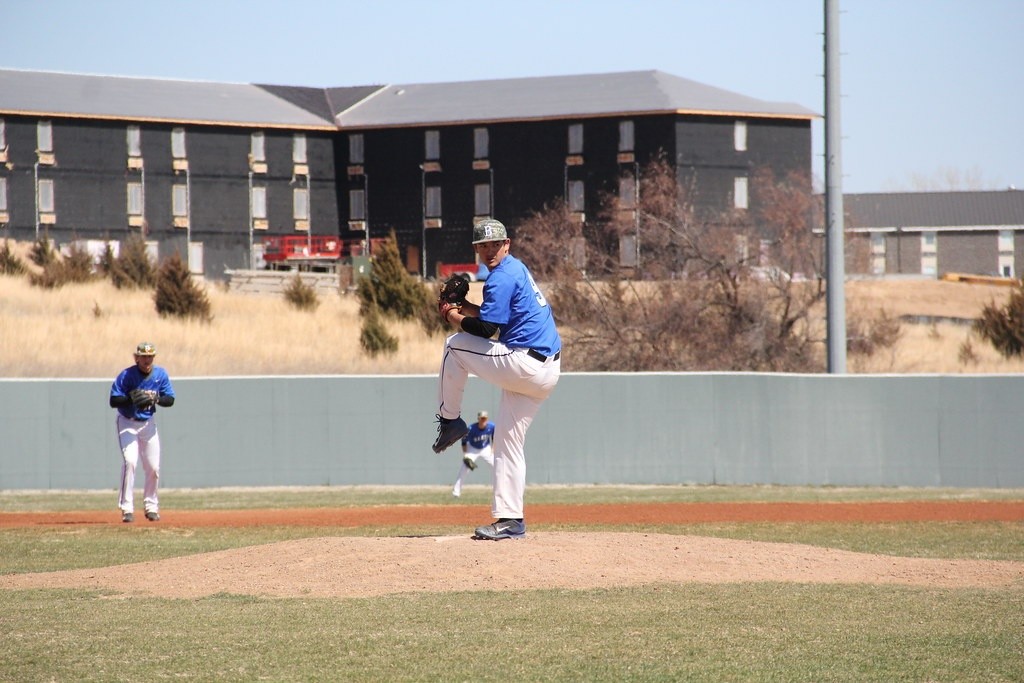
<box><xmin>144</xmin><ymin>512</ymin><xmax>159</xmax><ymax>522</ymax></box>
<box><xmin>122</xmin><ymin>513</ymin><xmax>133</xmax><ymax>521</ymax></box>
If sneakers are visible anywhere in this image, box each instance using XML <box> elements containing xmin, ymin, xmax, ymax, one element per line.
<box><xmin>431</xmin><ymin>414</ymin><xmax>468</xmax><ymax>454</ymax></box>
<box><xmin>475</xmin><ymin>516</ymin><xmax>526</xmax><ymax>540</ymax></box>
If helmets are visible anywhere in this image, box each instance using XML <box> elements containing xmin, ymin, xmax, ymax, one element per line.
<box><xmin>471</xmin><ymin>218</ymin><xmax>508</xmax><ymax>245</ymax></box>
<box><xmin>134</xmin><ymin>343</ymin><xmax>155</xmax><ymax>355</ymax></box>
<box><xmin>478</xmin><ymin>412</ymin><xmax>490</xmax><ymax>419</ymax></box>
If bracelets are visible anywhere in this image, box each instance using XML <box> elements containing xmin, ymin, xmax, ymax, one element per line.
<box><xmin>448</xmin><ymin>311</ymin><xmax>457</xmax><ymax>322</ymax></box>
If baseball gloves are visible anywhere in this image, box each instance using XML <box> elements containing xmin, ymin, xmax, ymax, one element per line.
<box><xmin>131</xmin><ymin>390</ymin><xmax>158</xmax><ymax>410</ymax></box>
<box><xmin>435</xmin><ymin>278</ymin><xmax>469</xmax><ymax>324</ymax></box>
<box><xmin>464</xmin><ymin>456</ymin><xmax>476</xmax><ymax>471</ymax></box>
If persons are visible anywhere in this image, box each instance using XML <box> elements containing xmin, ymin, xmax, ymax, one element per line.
<box><xmin>109</xmin><ymin>342</ymin><xmax>175</xmax><ymax>522</ymax></box>
<box><xmin>452</xmin><ymin>411</ymin><xmax>495</xmax><ymax>497</ymax></box>
<box><xmin>432</xmin><ymin>220</ymin><xmax>562</xmax><ymax>541</ymax></box>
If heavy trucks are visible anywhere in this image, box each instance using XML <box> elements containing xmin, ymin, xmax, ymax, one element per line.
<box><xmin>262</xmin><ymin>234</ymin><xmax>390</xmax><ymax>273</ymax></box>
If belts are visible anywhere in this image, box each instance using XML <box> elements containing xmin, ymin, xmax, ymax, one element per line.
<box><xmin>527</xmin><ymin>349</ymin><xmax>559</xmax><ymax>363</ymax></box>
<box><xmin>124</xmin><ymin>415</ymin><xmax>149</xmax><ymax>422</ymax></box>
